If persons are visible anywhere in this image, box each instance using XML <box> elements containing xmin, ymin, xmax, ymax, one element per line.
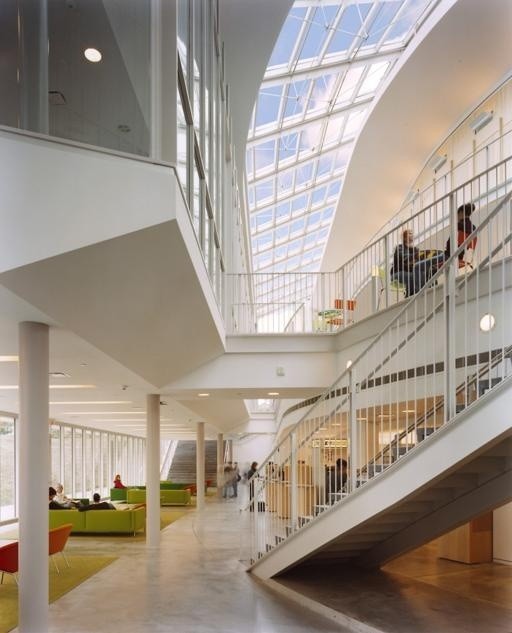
<box><xmin>388</xmin><ymin>226</ymin><xmax>428</xmax><ymax>300</ymax></box>
<box><xmin>75</xmin><ymin>491</ymin><xmax>117</xmax><ymax>511</ymax></box>
<box><xmin>55</xmin><ymin>483</ymin><xmax>75</xmax><ymax>509</ymax></box>
<box><xmin>433</xmin><ymin>202</ymin><xmax>478</xmax><ymax>272</ymax></box>
<box><xmin>324</xmin><ymin>456</ymin><xmax>349</xmax><ymax>491</ymax></box>
<box><xmin>114</xmin><ymin>473</ymin><xmax>127</xmax><ymax>489</ymax></box>
<box><xmin>222</xmin><ymin>460</ymin><xmax>287</xmax><ymax>517</ymax></box>
<box><xmin>50</xmin><ymin>486</ymin><xmax>68</xmax><ymax>510</ymax></box>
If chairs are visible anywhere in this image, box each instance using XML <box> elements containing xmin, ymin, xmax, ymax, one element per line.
<box><xmin>0</xmin><ymin>523</ymin><xmax>75</xmax><ymax>586</ymax></box>
<box><xmin>325</xmin><ymin>299</ymin><xmax>357</xmax><ymax>333</ymax></box>
<box><xmin>375</xmin><ymin>267</ymin><xmax>405</xmax><ymax>312</ymax></box>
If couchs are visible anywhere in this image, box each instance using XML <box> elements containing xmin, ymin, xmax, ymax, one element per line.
<box><xmin>48</xmin><ymin>498</ymin><xmax>146</xmax><ymax>537</ymax></box>
<box><xmin>110</xmin><ymin>481</ymin><xmax>191</xmax><ymax>506</ymax></box>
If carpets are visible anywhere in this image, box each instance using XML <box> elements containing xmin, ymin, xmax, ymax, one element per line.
<box><xmin>0</xmin><ymin>553</ymin><xmax>121</xmax><ymax>633</ymax></box>
<box><xmin>3</xmin><ymin>510</ymin><xmax>190</xmax><ymax>542</ymax></box>
